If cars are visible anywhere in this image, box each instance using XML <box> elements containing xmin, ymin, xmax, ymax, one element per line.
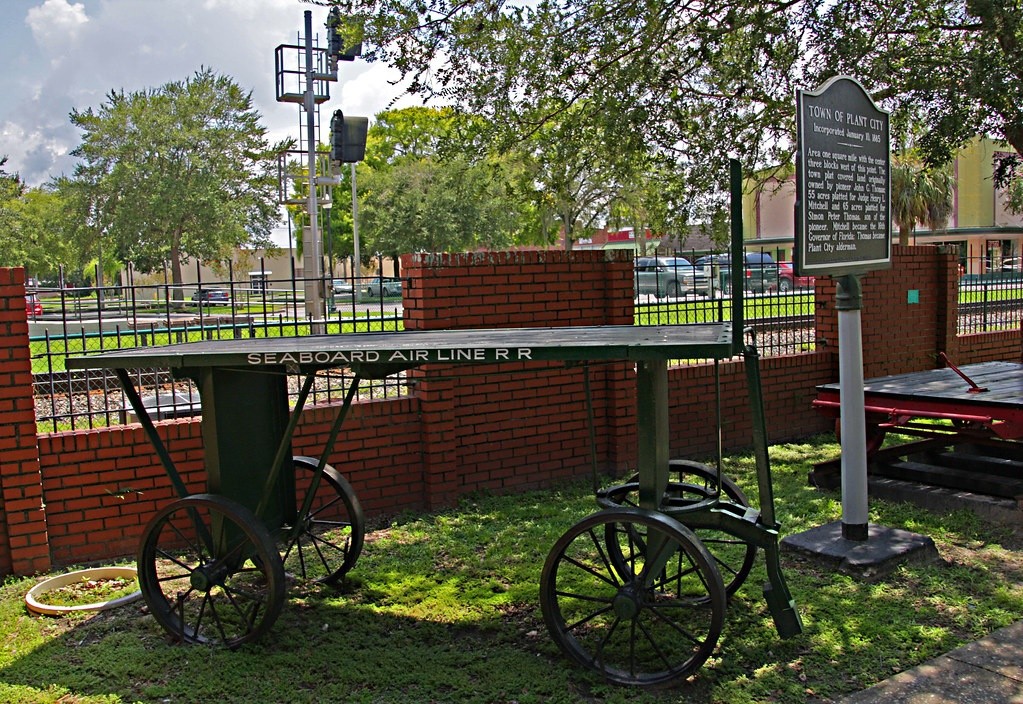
<box><xmin>25</xmin><ymin>295</ymin><xmax>43</xmax><ymax>316</ymax></box>
<box><xmin>191</xmin><ymin>287</ymin><xmax>229</xmax><ymax>308</ymax></box>
<box><xmin>367</xmin><ymin>278</ymin><xmax>402</xmax><ymax>297</ymax></box>
<box><xmin>333</xmin><ymin>280</ymin><xmax>352</xmax><ymax>294</ymax></box>
<box><xmin>766</xmin><ymin>261</ymin><xmax>815</xmax><ymax>292</ymax></box>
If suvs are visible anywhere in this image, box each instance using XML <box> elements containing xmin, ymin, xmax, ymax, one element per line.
<box><xmin>634</xmin><ymin>256</ymin><xmax>711</xmax><ymax>299</ymax></box>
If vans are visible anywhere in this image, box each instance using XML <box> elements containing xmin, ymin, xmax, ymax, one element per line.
<box><xmin>694</xmin><ymin>252</ymin><xmax>781</xmax><ymax>295</ymax></box>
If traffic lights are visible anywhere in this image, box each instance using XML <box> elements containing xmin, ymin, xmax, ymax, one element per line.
<box><xmin>330</xmin><ymin>109</ymin><xmax>368</xmax><ymax>162</ymax></box>
<box><xmin>327</xmin><ymin>6</ymin><xmax>364</xmax><ymax>61</ymax></box>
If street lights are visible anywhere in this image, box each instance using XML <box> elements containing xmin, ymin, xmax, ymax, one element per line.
<box><xmin>322</xmin><ymin>194</ymin><xmax>339</xmax><ymax>313</ymax></box>
